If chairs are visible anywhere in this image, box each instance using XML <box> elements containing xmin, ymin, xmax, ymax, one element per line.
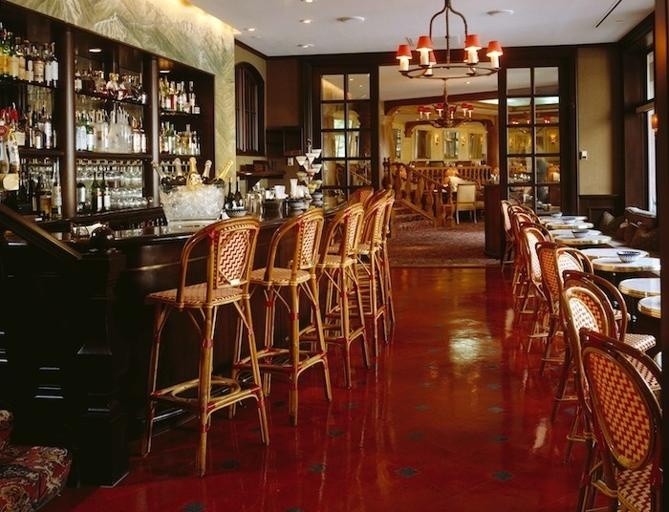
<box><xmin>0</xmin><ymin>405</ymin><xmax>71</xmax><ymax>512</ymax></box>
<box><xmin>456</xmin><ymin>182</ymin><xmax>479</xmax><ymax>224</ymax></box>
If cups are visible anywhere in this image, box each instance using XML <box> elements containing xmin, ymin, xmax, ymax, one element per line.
<box><xmin>245</xmin><ymin>190</ymin><xmax>275</xmax><ymax>218</ymax></box>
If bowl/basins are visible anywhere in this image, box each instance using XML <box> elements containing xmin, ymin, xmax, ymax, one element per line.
<box><xmin>540</xmin><ymin>203</ymin><xmax>551</xmax><ymax>210</ymax></box>
<box><xmin>157</xmin><ymin>186</ymin><xmax>223</xmax><ymax>223</ymax></box>
<box><xmin>288</xmin><ymin>201</ymin><xmax>306</xmax><ymax>210</ymax></box>
<box><xmin>225</xmin><ymin>208</ymin><xmax>251</xmax><ymax>217</ymax></box>
<box><xmin>311</xmin><ymin>193</ymin><xmax>324</xmax><ymax>202</ymax></box>
<box><xmin>551</xmin><ymin>210</ymin><xmax>640</xmax><ymax>263</ymax></box>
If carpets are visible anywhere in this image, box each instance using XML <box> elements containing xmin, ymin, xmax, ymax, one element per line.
<box><xmin>388</xmin><ymin>204</ymin><xmax>499</xmax><ymax>267</ymax></box>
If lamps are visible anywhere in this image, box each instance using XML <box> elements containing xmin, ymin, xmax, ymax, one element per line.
<box><xmin>512</xmin><ymin>112</ymin><xmax>550</xmax><ymax>136</ymax></box>
<box><xmin>418</xmin><ymin>77</ymin><xmax>473</xmax><ymax>129</ymax></box>
<box><xmin>395</xmin><ymin>0</ymin><xmax>503</xmax><ymax>79</ymax></box>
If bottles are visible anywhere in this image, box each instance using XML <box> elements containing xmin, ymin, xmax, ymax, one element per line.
<box><xmin>159</xmin><ymin>76</ymin><xmax>199</xmax><ymax>155</ymax></box>
<box><xmin>1</xmin><ymin>24</ymin><xmax>58</xmax><ymax>87</ymax></box>
<box><xmin>74</xmin><ymin>47</ymin><xmax>147</xmax><ymax>211</ymax></box>
<box><xmin>17</xmin><ymin>157</ymin><xmax>62</xmax><ymax>214</ymax></box>
<box><xmin>226</xmin><ymin>176</ymin><xmax>243</xmax><ymax>207</ymax></box>
<box><xmin>111</xmin><ymin>215</ymin><xmax>167</xmax><ymax>237</ymax></box>
<box><xmin>151</xmin><ymin>156</ymin><xmax>233</xmax><ymax>193</ymax></box>
<box><xmin>8</xmin><ymin>102</ymin><xmax>56</xmax><ymax>150</ymax></box>
